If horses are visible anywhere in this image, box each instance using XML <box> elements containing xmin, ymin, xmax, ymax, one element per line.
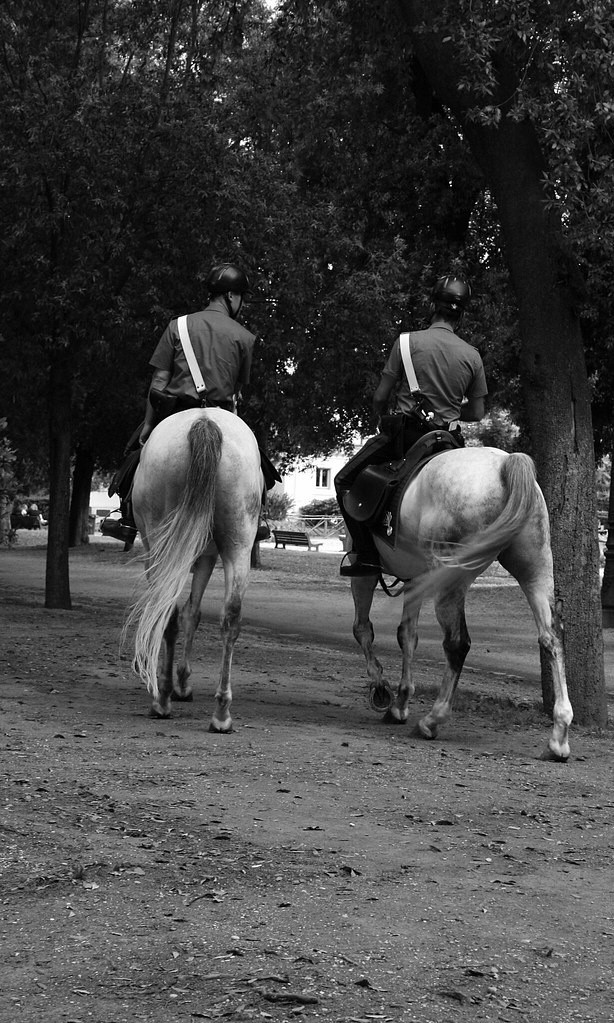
<box><xmin>117</xmin><ymin>407</ymin><xmax>266</xmax><ymax>734</ymax></box>
<box><xmin>343</xmin><ymin>446</ymin><xmax>574</xmax><ymax>764</ymax></box>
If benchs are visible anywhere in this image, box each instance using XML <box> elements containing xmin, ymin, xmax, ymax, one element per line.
<box><xmin>271</xmin><ymin>530</ymin><xmax>322</xmax><ymax>552</ymax></box>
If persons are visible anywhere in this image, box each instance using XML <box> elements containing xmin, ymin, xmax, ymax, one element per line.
<box><xmin>99</xmin><ymin>263</ymin><xmax>270</xmax><ymax>541</ymax></box>
<box><xmin>11</xmin><ymin>503</ymin><xmax>47</xmax><ymax>530</ymax></box>
<box><xmin>334</xmin><ymin>276</ymin><xmax>488</xmax><ymax>575</ymax></box>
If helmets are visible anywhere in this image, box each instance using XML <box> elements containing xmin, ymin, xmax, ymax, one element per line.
<box><xmin>204</xmin><ymin>262</ymin><xmax>255</xmax><ymax>295</ymax></box>
<box><xmin>432</xmin><ymin>275</ymin><xmax>472</xmax><ymax>309</ymax></box>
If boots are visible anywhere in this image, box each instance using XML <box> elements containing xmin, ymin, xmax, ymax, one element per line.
<box><xmin>339</xmin><ymin>490</ymin><xmax>378</xmax><ymax>576</ymax></box>
<box><xmin>101</xmin><ymin>495</ymin><xmax>137</xmax><ymax>543</ymax></box>
